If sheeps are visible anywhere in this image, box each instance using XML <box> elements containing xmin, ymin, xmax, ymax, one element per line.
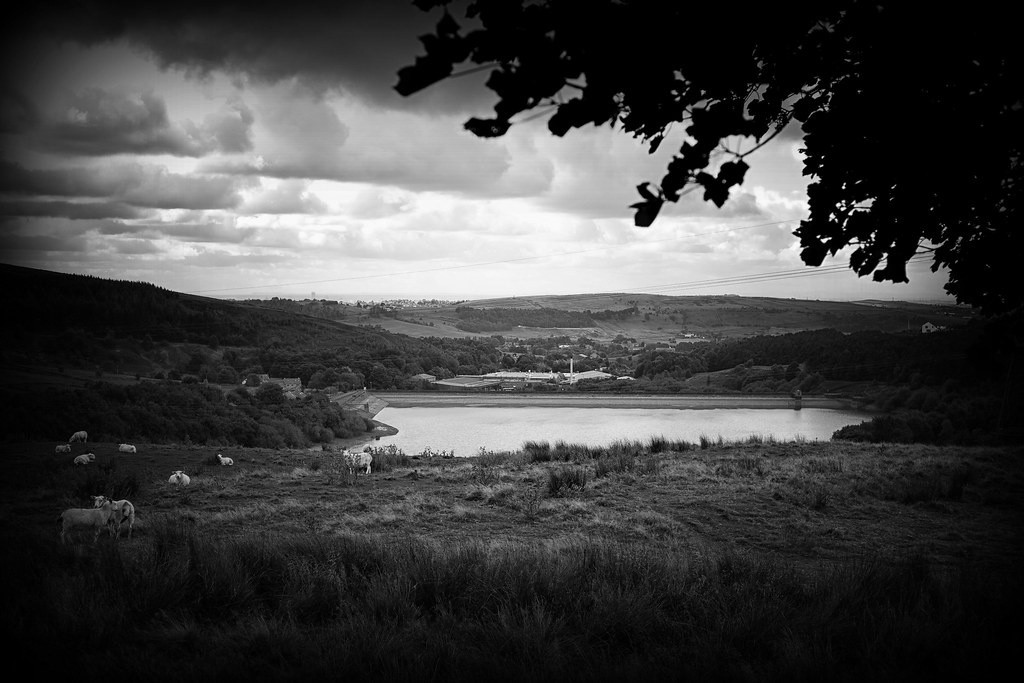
<box><xmin>68</xmin><ymin>431</ymin><xmax>87</xmax><ymax>443</ymax></box>
<box><xmin>169</xmin><ymin>470</ymin><xmax>189</xmax><ymax>486</ymax></box>
<box><xmin>340</xmin><ymin>448</ymin><xmax>372</xmax><ymax>475</ymax></box>
<box><xmin>55</xmin><ymin>444</ymin><xmax>71</xmax><ymax>453</ymax></box>
<box><xmin>73</xmin><ymin>452</ymin><xmax>95</xmax><ymax>466</ymax></box>
<box><xmin>217</xmin><ymin>454</ymin><xmax>234</xmax><ymax>466</ymax></box>
<box><xmin>57</xmin><ymin>495</ymin><xmax>135</xmax><ymax>545</ymax></box>
<box><xmin>119</xmin><ymin>443</ymin><xmax>136</xmax><ymax>453</ymax></box>
